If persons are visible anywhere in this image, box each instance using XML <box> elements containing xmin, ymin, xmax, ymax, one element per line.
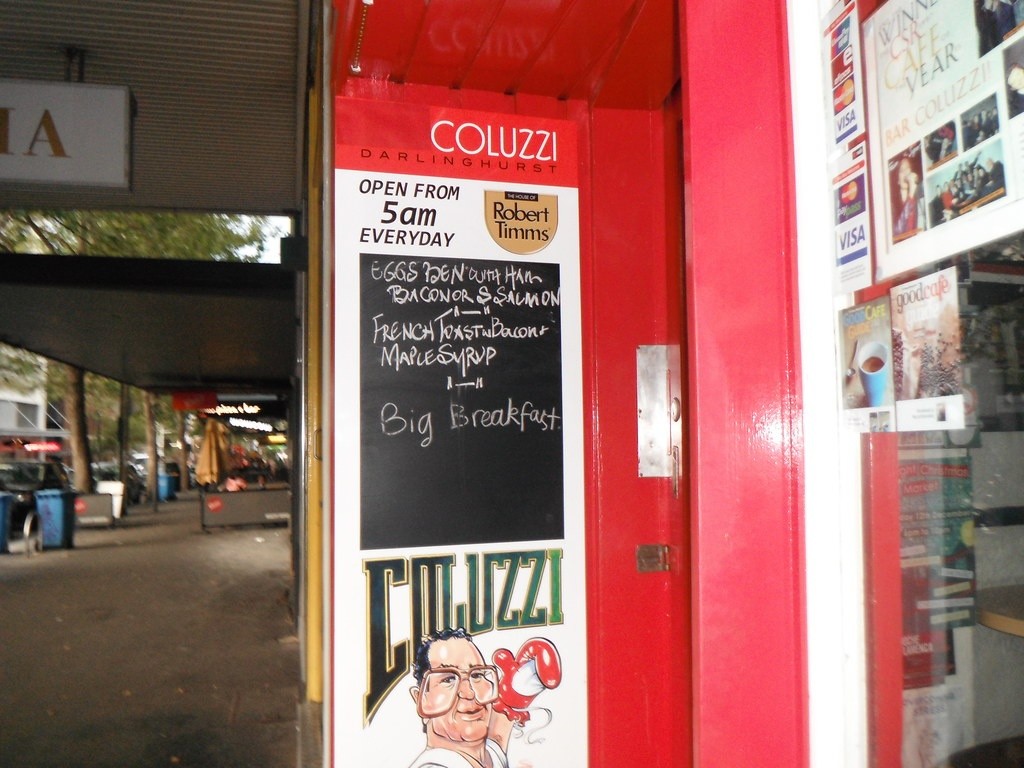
<box><xmin>962</xmin><ymin>107</ymin><xmax>998</xmax><ymax>151</ymax></box>
<box><xmin>935</xmin><ymin>137</ymin><xmax>951</xmax><ymax>164</ymax></box>
<box><xmin>216</xmin><ymin>470</ymin><xmax>248</xmax><ymax>492</ymax></box>
<box><xmin>930</xmin><ymin>151</ymin><xmax>1005</xmax><ymax>227</ymax></box>
<box><xmin>1007</xmin><ymin>64</ymin><xmax>1024</xmax><ymax>114</ymax></box>
<box><xmin>892</xmin><ymin>157</ymin><xmax>925</xmax><ymax>234</ymax></box>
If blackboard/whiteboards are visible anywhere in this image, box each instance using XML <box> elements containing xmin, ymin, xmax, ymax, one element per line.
<box><xmin>358</xmin><ymin>251</ymin><xmax>566</xmax><ymax>551</ymax></box>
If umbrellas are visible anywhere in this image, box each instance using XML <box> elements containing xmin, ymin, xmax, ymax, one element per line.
<box><xmin>194</xmin><ymin>418</ymin><xmax>233</xmax><ymax>485</ymax></box>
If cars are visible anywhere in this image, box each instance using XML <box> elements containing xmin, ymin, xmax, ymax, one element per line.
<box><xmin>0</xmin><ymin>459</ymin><xmax>70</xmax><ymax>529</ymax></box>
<box><xmin>165</xmin><ymin>462</ymin><xmax>190</xmax><ymax>490</ymax></box>
<box><xmin>88</xmin><ymin>461</ymin><xmax>146</xmax><ymax>505</ymax></box>
<box><xmin>244</xmin><ymin>454</ymin><xmax>273</xmax><ymax>478</ymax></box>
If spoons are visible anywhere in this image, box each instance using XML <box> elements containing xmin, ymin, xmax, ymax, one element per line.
<box><xmin>846</xmin><ymin>340</ymin><xmax>859</xmax><ymax>376</ymax></box>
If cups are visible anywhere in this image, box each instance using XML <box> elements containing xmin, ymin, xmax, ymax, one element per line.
<box><xmin>869</xmin><ymin>412</ymin><xmax>877</xmax><ymax>433</ymax></box>
<box><xmin>858</xmin><ymin>342</ymin><xmax>888</xmax><ymax>406</ymax></box>
<box><xmin>878</xmin><ymin>412</ymin><xmax>889</xmax><ymax>432</ymax></box>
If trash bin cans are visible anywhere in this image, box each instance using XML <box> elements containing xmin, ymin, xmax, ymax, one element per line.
<box><xmin>0</xmin><ymin>491</ymin><xmax>15</xmax><ymax>555</ymax></box>
<box><xmin>33</xmin><ymin>488</ymin><xmax>76</xmax><ymax>553</ymax></box>
<box><xmin>158</xmin><ymin>472</ymin><xmax>180</xmax><ymax>503</ymax></box>
